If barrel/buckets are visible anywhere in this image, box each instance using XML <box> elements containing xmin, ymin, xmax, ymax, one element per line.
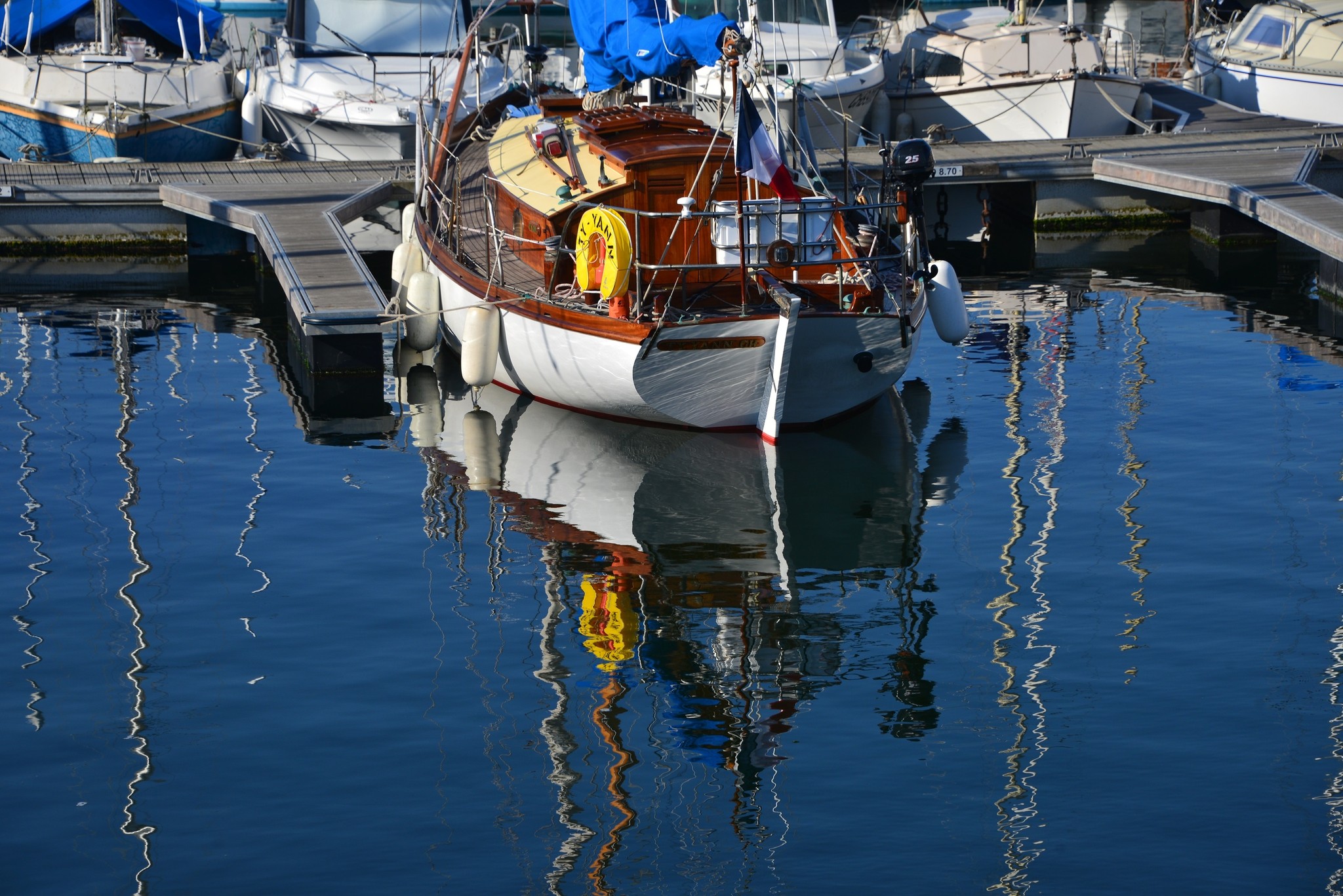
<box><xmin>119</xmin><ymin>36</ymin><xmax>147</xmax><ymax>62</ymax></box>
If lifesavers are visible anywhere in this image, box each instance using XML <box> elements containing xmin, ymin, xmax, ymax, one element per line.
<box><xmin>579</xmin><ymin>571</ymin><xmax>639</xmax><ymax>671</ymax></box>
<box><xmin>574</xmin><ymin>205</ymin><xmax>633</xmax><ymax>299</ymax></box>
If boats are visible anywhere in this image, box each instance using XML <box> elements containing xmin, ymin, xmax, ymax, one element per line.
<box><xmin>1189</xmin><ymin>1</ymin><xmax>1343</xmax><ymax>126</ymax></box>
<box><xmin>410</xmin><ymin>0</ymin><xmax>929</xmax><ymax>446</ymax></box>
<box><xmin>1</xmin><ymin>0</ymin><xmax>1145</xmax><ymax>163</ymax></box>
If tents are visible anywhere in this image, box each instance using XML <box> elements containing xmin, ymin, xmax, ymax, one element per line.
<box><xmin>0</xmin><ymin>0</ymin><xmax>226</xmax><ymax>61</ymax></box>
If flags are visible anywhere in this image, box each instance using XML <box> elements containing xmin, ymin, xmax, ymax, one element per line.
<box><xmin>734</xmin><ymin>77</ymin><xmax>801</xmax><ymax>201</ymax></box>
